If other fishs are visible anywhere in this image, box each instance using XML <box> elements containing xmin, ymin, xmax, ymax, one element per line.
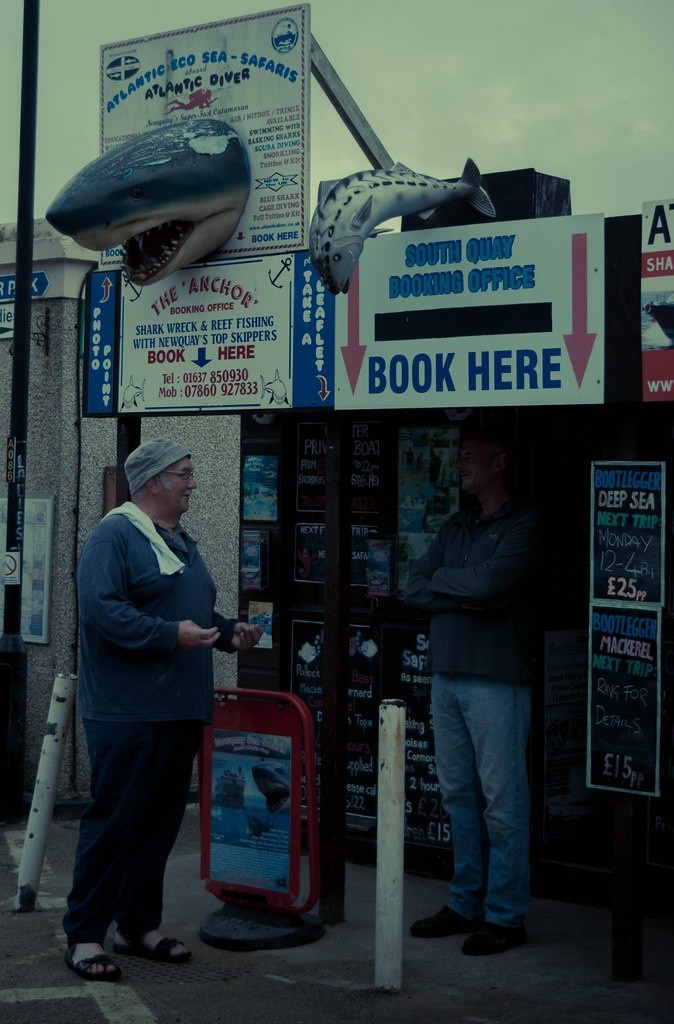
<box><xmin>306</xmin><ymin>154</ymin><xmax>499</xmax><ymax>296</ymax></box>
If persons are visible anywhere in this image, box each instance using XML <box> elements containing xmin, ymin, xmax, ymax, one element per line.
<box><xmin>64</xmin><ymin>437</ymin><xmax>263</xmax><ymax>981</ymax></box>
<box><xmin>405</xmin><ymin>431</ymin><xmax>545</xmax><ymax>958</ymax></box>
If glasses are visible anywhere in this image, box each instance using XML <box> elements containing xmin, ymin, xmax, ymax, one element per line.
<box><xmin>165</xmin><ymin>470</ymin><xmax>193</xmax><ymax>479</ymax></box>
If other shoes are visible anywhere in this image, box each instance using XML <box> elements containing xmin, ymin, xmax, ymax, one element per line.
<box><xmin>462</xmin><ymin>922</ymin><xmax>527</xmax><ymax>956</ymax></box>
<box><xmin>409</xmin><ymin>906</ymin><xmax>469</xmax><ymax>938</ymax></box>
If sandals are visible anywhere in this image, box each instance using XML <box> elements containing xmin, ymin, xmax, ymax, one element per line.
<box><xmin>112</xmin><ymin>927</ymin><xmax>193</xmax><ymax>963</ymax></box>
<box><xmin>65</xmin><ymin>943</ymin><xmax>122</xmax><ymax>981</ymax></box>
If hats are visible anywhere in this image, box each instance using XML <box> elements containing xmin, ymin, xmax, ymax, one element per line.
<box><xmin>124</xmin><ymin>437</ymin><xmax>191</xmax><ymax>495</ymax></box>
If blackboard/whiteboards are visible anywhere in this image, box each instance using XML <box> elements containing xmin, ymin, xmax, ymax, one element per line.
<box><xmin>585</xmin><ymin>601</ymin><xmax>661</xmax><ymax>797</ymax></box>
<box><xmin>588</xmin><ymin>459</ymin><xmax>666</xmax><ymax>609</ymax></box>
